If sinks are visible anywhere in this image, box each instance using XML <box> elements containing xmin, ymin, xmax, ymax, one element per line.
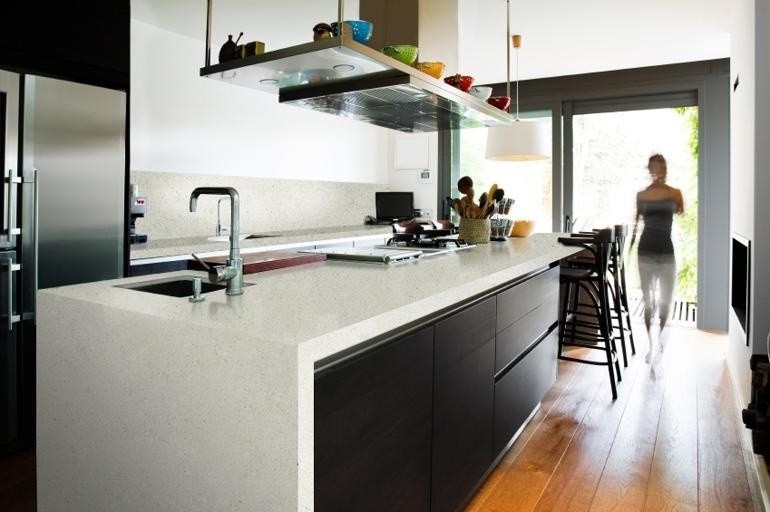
<box><xmin>112</xmin><ymin>273</ymin><xmax>257</xmax><ymax>299</ymax></box>
<box><xmin>206</xmin><ymin>232</ymin><xmax>283</xmax><ymax>242</ymax></box>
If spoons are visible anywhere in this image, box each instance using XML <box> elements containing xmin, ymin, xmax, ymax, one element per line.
<box><xmin>447</xmin><ymin>176</ymin><xmax>502</xmax><ymax>218</ymax></box>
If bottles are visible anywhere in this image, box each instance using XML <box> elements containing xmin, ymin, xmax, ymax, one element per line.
<box><xmin>218</xmin><ymin>34</ymin><xmax>237</xmax><ymax>61</ymax></box>
<box><xmin>493</xmin><ymin>197</ymin><xmax>515</xmax><ymax>218</ymax></box>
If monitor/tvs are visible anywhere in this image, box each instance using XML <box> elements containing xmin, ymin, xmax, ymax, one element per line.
<box><xmin>375</xmin><ymin>191</ymin><xmax>413</xmax><ymax>222</ymax></box>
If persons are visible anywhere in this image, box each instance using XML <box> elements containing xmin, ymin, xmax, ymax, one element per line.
<box><xmin>626</xmin><ymin>153</ymin><xmax>686</xmax><ymax>363</ymax></box>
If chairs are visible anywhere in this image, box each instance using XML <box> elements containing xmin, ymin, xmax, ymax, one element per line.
<box><xmin>557</xmin><ymin>222</ymin><xmax>636</xmax><ymax>400</ymax></box>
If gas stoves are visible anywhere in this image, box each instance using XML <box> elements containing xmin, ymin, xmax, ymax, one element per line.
<box><xmin>375</xmin><ymin>226</ymin><xmax>467</xmax><ymax>250</ymax></box>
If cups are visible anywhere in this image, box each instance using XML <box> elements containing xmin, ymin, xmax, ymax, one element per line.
<box><xmin>130</xmin><ymin>184</ymin><xmax>139</xmax><ymax>196</ymax></box>
<box><xmin>460</xmin><ymin>219</ymin><xmax>490</xmax><ymax>245</ymax></box>
<box><xmin>236</xmin><ymin>41</ymin><xmax>265</xmax><ymax>59</ymax></box>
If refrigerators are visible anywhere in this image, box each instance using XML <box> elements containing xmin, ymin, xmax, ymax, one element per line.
<box><xmin>2</xmin><ymin>0</ymin><xmax>128</xmax><ymax>511</ymax></box>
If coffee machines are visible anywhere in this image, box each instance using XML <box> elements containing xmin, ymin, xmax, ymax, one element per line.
<box><xmin>129</xmin><ymin>195</ymin><xmax>149</xmax><ymax>245</ymax></box>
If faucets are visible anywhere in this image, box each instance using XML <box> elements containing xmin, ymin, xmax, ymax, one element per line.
<box><xmin>216</xmin><ymin>196</ymin><xmax>231</xmax><ymax>236</ymax></box>
<box><xmin>187</xmin><ymin>186</ymin><xmax>244</xmax><ymax>297</ymax></box>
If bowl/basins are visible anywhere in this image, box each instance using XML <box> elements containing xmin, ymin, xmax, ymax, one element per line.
<box><xmin>331</xmin><ymin>19</ymin><xmax>374</xmax><ymax>44</ymax></box>
<box><xmin>382</xmin><ymin>44</ymin><xmax>446</xmax><ymax>79</ymax></box>
<box><xmin>490</xmin><ymin>219</ymin><xmax>532</xmax><ymax>240</ymax></box>
<box><xmin>444</xmin><ymin>75</ymin><xmax>510</xmax><ymax>111</ymax></box>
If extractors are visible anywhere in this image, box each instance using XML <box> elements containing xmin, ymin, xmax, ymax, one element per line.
<box><xmin>198</xmin><ymin>1</ymin><xmax>516</xmax><ymax>133</ymax></box>
